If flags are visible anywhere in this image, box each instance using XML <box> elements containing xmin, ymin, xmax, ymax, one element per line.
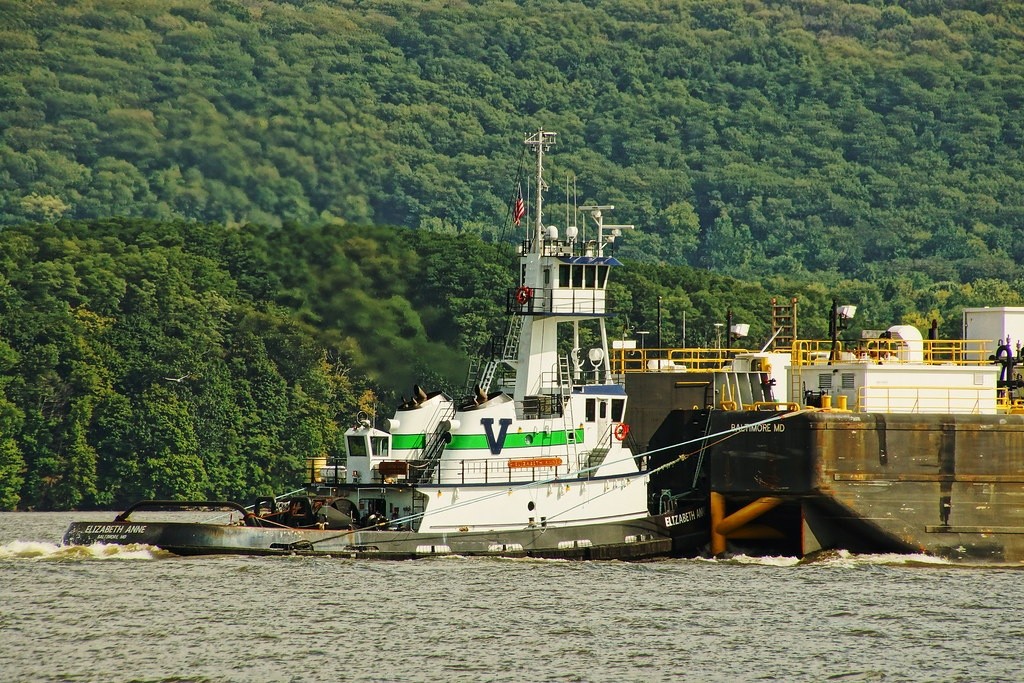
<box><xmin>513</xmin><ymin>181</ymin><xmax>524</xmax><ymax>226</ymax></box>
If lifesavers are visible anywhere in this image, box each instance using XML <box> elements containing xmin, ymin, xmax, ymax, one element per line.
<box><xmin>516</xmin><ymin>286</ymin><xmax>531</xmax><ymax>304</ymax></box>
<box><xmin>615</xmin><ymin>423</ymin><xmax>629</xmax><ymax>442</ymax></box>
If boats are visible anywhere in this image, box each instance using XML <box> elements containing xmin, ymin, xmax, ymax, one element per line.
<box><xmin>645</xmin><ymin>299</ymin><xmax>1024</xmax><ymax>571</ymax></box>
<box><xmin>60</xmin><ymin>126</ymin><xmax>711</xmax><ymax>565</ymax></box>
